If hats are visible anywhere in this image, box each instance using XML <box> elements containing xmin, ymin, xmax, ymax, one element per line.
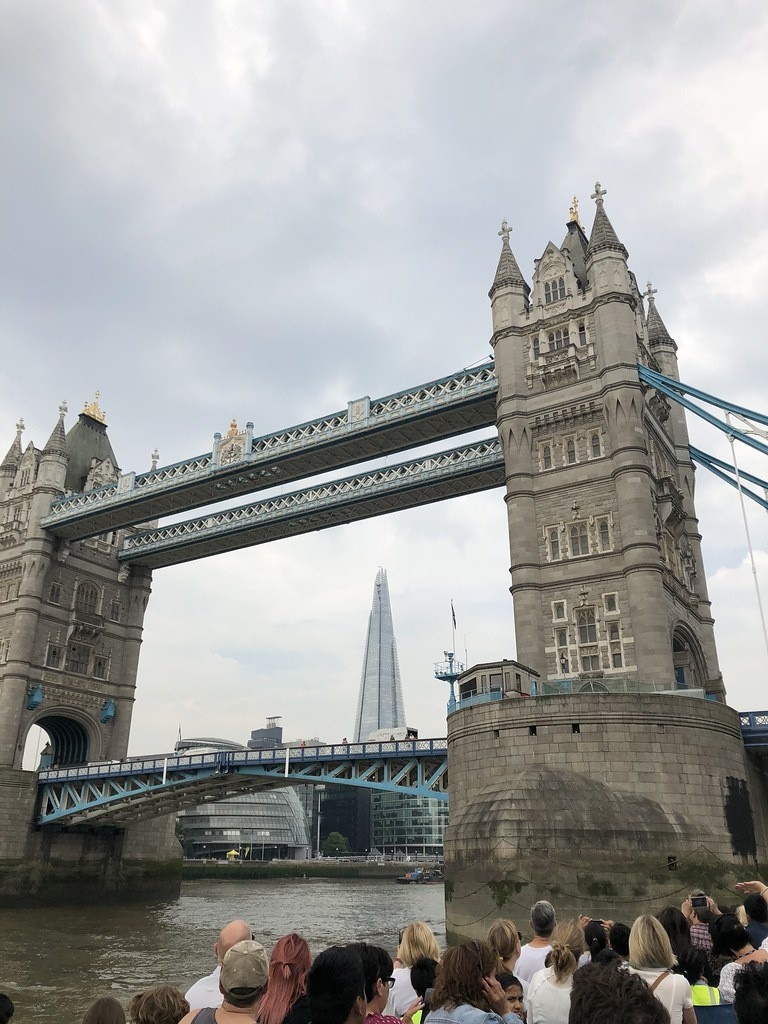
<box><xmin>220</xmin><ymin>939</ymin><xmax>269</xmax><ymax>1001</ymax></box>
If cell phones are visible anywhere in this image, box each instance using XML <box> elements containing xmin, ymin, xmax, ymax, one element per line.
<box><xmin>482</xmin><ymin>982</ymin><xmax>491</xmax><ymax>990</ymax></box>
<box><xmin>591</xmin><ymin>920</ymin><xmax>604</xmax><ymax>926</ymax></box>
<box><xmin>690</xmin><ymin>897</ymin><xmax>709</xmax><ymax>908</ymax></box>
<box><xmin>424</xmin><ymin>988</ymin><xmax>435</xmax><ymax>1003</ymax></box>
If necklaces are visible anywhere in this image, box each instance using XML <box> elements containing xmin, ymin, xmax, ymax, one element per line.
<box><xmin>739</xmin><ymin>949</ymin><xmax>755</xmax><ymax>958</ymax></box>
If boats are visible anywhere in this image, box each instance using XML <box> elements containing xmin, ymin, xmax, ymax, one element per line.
<box><xmin>395</xmin><ymin>868</ymin><xmax>430</xmax><ymax>885</ymax></box>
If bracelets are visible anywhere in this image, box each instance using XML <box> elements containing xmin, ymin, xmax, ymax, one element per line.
<box><xmin>395</xmin><ymin>958</ymin><xmax>403</xmax><ymax>964</ymax></box>
<box><xmin>761</xmin><ymin>887</ymin><xmax>768</xmax><ymax>895</ymax></box>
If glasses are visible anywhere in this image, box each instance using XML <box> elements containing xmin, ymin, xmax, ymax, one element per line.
<box><xmin>512</xmin><ymin>931</ymin><xmax>522</xmax><ymax>948</ymax></box>
<box><xmin>252</xmin><ymin>934</ymin><xmax>256</xmax><ymax>942</ymax></box>
<box><xmin>380</xmin><ymin>977</ymin><xmax>396</xmax><ymax>988</ymax></box>
<box><xmin>470</xmin><ymin>939</ymin><xmax>483</xmax><ymax>977</ymax></box>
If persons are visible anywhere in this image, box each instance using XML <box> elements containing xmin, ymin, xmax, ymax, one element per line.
<box><xmin>0</xmin><ymin>993</ymin><xmax>14</xmax><ymax>1024</ymax></box>
<box><xmin>84</xmin><ymin>881</ymin><xmax>768</xmax><ymax>1024</ymax></box>
